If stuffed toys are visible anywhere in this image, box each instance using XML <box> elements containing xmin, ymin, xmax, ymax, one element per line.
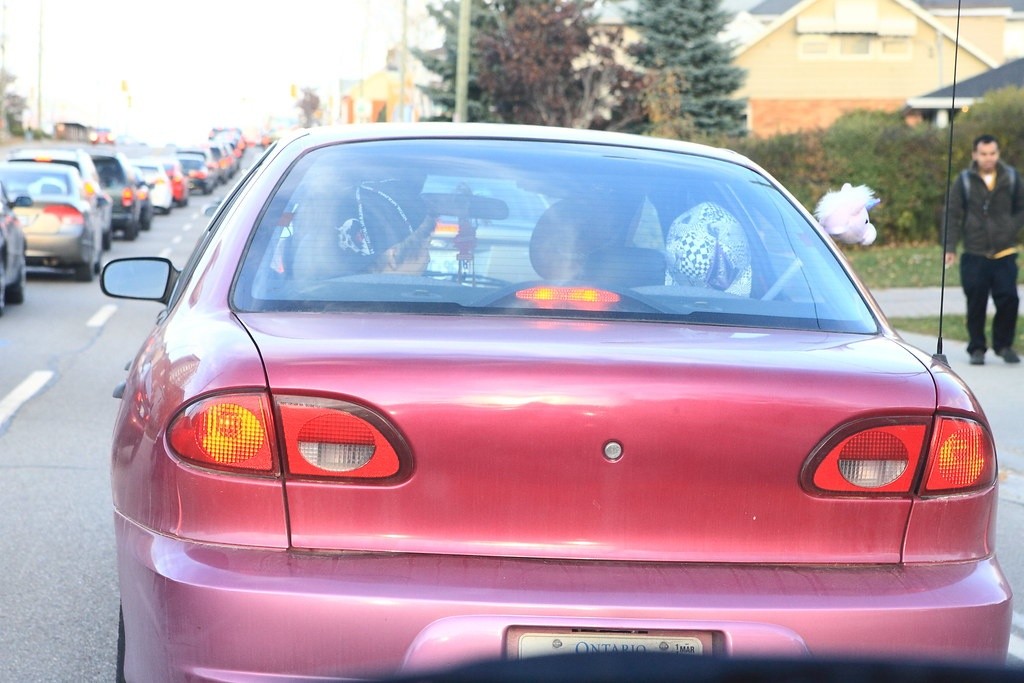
<box><xmin>814</xmin><ymin>183</ymin><xmax>882</xmax><ymax>246</ymax></box>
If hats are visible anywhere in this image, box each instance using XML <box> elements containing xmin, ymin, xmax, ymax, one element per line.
<box><xmin>321</xmin><ymin>177</ymin><xmax>427</xmax><ymax>274</ymax></box>
<box><xmin>666</xmin><ymin>202</ymin><xmax>752</xmax><ymax>298</ymax></box>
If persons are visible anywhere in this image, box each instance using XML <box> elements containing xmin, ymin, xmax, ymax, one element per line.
<box><xmin>336</xmin><ymin>177</ymin><xmax>439</xmax><ymax>276</ymax></box>
<box><xmin>941</xmin><ymin>133</ymin><xmax>1024</xmax><ymax>364</ymax></box>
<box><xmin>659</xmin><ymin>201</ymin><xmax>757</xmax><ymax>296</ymax></box>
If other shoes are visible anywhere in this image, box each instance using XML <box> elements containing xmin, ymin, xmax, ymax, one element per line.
<box><xmin>995</xmin><ymin>346</ymin><xmax>1020</xmax><ymax>363</ymax></box>
<box><xmin>970</xmin><ymin>348</ymin><xmax>986</xmax><ymax>365</ymax></box>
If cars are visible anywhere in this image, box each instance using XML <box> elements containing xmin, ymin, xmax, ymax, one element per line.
<box><xmin>257</xmin><ymin>170</ymin><xmax>575</xmax><ymax>293</ymax></box>
<box><xmin>99</xmin><ymin>116</ymin><xmax>1016</xmax><ymax>683</ymax></box>
<box><xmin>1</xmin><ymin>119</ymin><xmax>245</xmax><ymax>324</ymax></box>
